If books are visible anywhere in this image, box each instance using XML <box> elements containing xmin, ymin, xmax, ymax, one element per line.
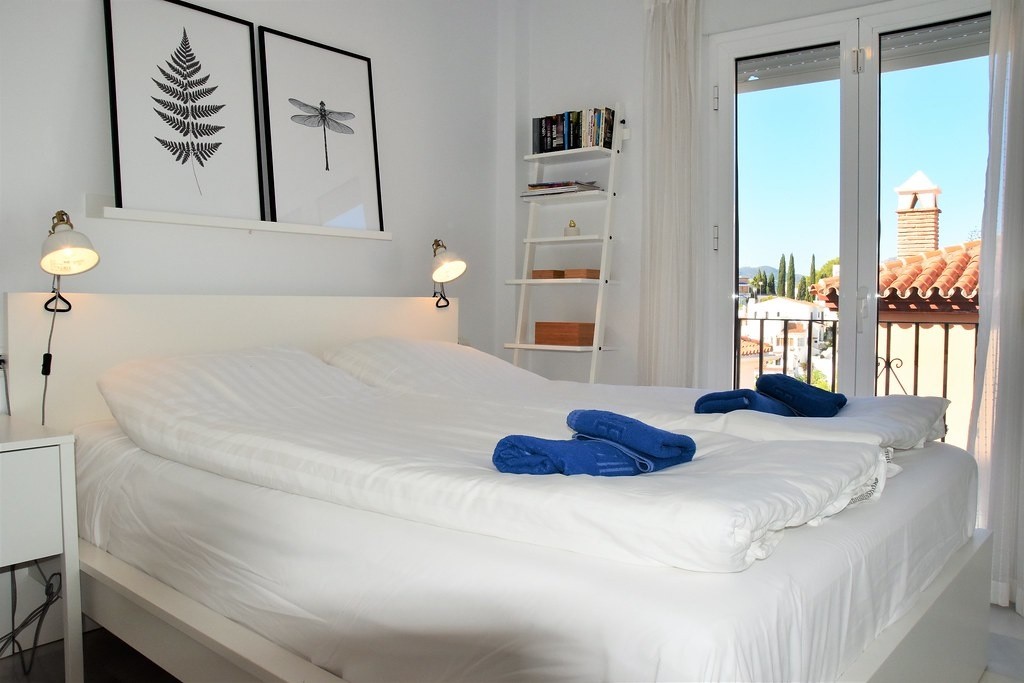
<box><xmin>532</xmin><ymin>106</ymin><xmax>615</xmax><ymax>154</ymax></box>
<box><xmin>520</xmin><ymin>180</ymin><xmax>605</xmax><ymax>197</ymax></box>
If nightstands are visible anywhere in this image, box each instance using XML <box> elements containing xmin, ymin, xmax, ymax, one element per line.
<box><xmin>0</xmin><ymin>409</ymin><xmax>85</xmax><ymax>683</ymax></box>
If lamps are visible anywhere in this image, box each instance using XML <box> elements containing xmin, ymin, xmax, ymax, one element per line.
<box><xmin>430</xmin><ymin>239</ymin><xmax>467</xmax><ymax>309</ymax></box>
<box><xmin>40</xmin><ymin>210</ymin><xmax>100</xmax><ymax>312</ymax></box>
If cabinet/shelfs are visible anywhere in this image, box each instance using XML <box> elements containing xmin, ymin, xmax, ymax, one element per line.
<box><xmin>504</xmin><ymin>103</ymin><xmax>632</xmax><ymax>386</ymax></box>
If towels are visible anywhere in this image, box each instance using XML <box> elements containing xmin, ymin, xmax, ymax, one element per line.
<box><xmin>694</xmin><ymin>374</ymin><xmax>848</xmax><ymax>418</ymax></box>
<box><xmin>493</xmin><ymin>407</ymin><xmax>696</xmax><ymax>476</ymax></box>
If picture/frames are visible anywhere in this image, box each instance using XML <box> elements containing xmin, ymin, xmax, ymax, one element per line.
<box><xmin>258</xmin><ymin>24</ymin><xmax>385</xmax><ymax>232</ymax></box>
<box><xmin>105</xmin><ymin>0</ymin><xmax>265</xmax><ymax>222</ymax></box>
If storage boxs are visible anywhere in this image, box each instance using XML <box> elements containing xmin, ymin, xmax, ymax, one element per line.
<box><xmin>535</xmin><ymin>322</ymin><xmax>596</xmax><ymax>346</ymax></box>
<box><xmin>564</xmin><ymin>268</ymin><xmax>599</xmax><ymax>279</ymax></box>
<box><xmin>532</xmin><ymin>270</ymin><xmax>565</xmax><ymax>278</ymax></box>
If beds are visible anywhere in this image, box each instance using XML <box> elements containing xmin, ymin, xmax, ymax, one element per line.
<box><xmin>3</xmin><ymin>291</ymin><xmax>995</xmax><ymax>683</ymax></box>
